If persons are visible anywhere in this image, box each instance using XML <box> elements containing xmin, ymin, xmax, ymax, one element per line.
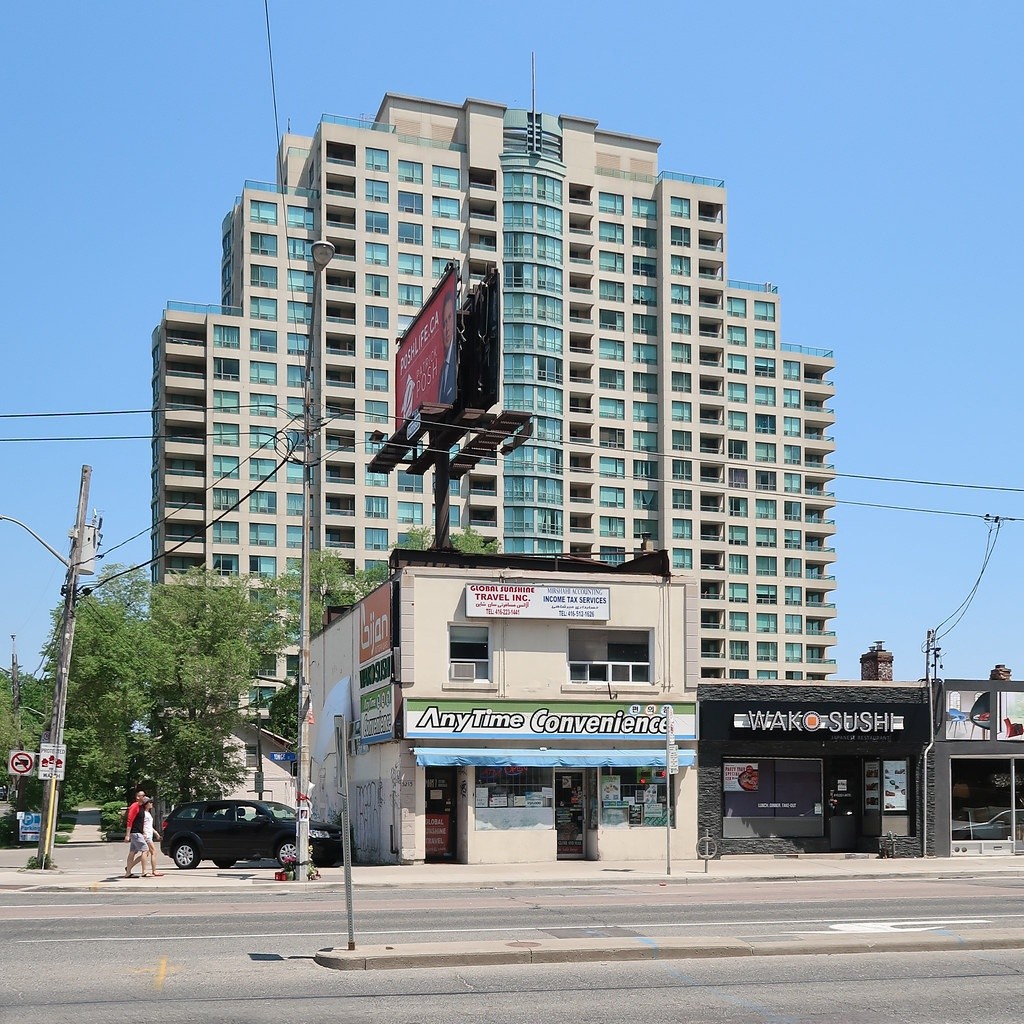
<box><xmin>123</xmin><ymin>791</ymin><xmax>155</xmax><ymax>878</ymax></box>
<box><xmin>125</xmin><ymin>797</ymin><xmax>165</xmax><ymax>876</ymax></box>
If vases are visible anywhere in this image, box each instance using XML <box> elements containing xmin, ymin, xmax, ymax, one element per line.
<box><xmin>285</xmin><ymin>871</ymin><xmax>294</xmax><ymax>881</ymax></box>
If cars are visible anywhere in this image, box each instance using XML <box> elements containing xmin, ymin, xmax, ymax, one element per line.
<box><xmin>952</xmin><ymin>809</ymin><xmax>1024</xmax><ymax>842</ymax></box>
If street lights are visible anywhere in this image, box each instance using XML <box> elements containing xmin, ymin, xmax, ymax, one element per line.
<box><xmin>294</xmin><ymin>240</ymin><xmax>336</xmax><ymax>880</ymax></box>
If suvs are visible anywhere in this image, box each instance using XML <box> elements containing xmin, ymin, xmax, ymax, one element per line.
<box><xmin>161</xmin><ymin>800</ymin><xmax>344</xmax><ymax>872</ymax></box>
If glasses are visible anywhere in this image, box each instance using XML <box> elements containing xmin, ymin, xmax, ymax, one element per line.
<box><xmin>137</xmin><ymin>794</ymin><xmax>147</xmax><ymax>797</ymax></box>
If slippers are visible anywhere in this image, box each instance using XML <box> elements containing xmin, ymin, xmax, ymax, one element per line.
<box><xmin>125</xmin><ymin>874</ymin><xmax>139</xmax><ymax>878</ymax></box>
<box><xmin>141</xmin><ymin>871</ymin><xmax>164</xmax><ymax>877</ymax></box>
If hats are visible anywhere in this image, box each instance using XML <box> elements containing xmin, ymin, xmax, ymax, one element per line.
<box><xmin>143</xmin><ymin>796</ymin><xmax>152</xmax><ymax>804</ymax></box>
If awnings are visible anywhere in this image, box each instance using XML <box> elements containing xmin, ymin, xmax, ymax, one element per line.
<box><xmin>408</xmin><ymin>746</ymin><xmax>698</xmax><ymax>767</ymax></box>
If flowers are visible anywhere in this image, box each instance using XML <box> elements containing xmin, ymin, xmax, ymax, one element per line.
<box><xmin>283</xmin><ymin>856</ymin><xmax>296</xmax><ymax>872</ymax></box>
